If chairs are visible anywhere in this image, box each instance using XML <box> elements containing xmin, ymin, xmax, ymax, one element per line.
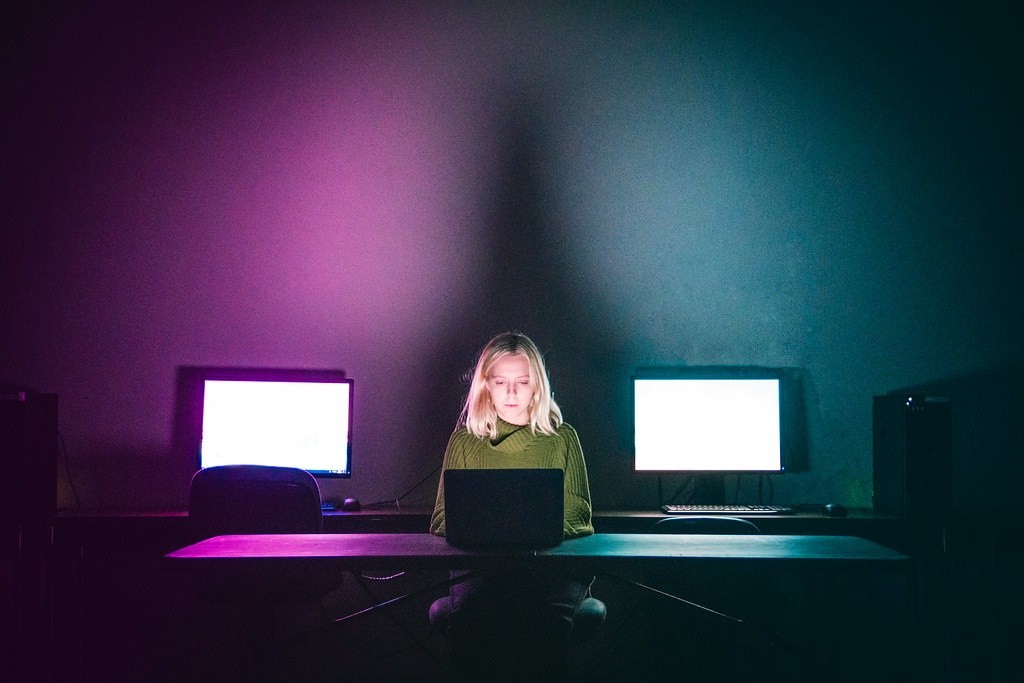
<box><xmin>186</xmin><ymin>465</ymin><xmax>344</xmax><ymax>683</ymax></box>
<box><xmin>652</xmin><ymin>517</ymin><xmax>789</xmax><ymax>683</ymax></box>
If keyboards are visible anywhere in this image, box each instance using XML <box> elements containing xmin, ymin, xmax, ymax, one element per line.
<box><xmin>661</xmin><ymin>506</ymin><xmax>779</xmax><ymax>515</ymax></box>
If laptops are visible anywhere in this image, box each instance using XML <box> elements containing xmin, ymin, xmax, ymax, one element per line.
<box><xmin>444</xmin><ymin>468</ymin><xmax>565</xmax><ymax>547</ymax></box>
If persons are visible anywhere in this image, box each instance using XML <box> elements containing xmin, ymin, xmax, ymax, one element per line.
<box><xmin>428</xmin><ymin>333</ymin><xmax>605</xmax><ymax>645</ymax></box>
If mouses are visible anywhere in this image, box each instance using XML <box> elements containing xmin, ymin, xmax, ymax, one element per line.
<box><xmin>822</xmin><ymin>503</ymin><xmax>847</xmax><ymax>517</ymax></box>
<box><xmin>343</xmin><ymin>499</ymin><xmax>360</xmax><ymax>511</ymax></box>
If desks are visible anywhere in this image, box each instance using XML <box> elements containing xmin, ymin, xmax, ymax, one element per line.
<box><xmin>163</xmin><ymin>534</ymin><xmax>912</xmax><ymax>683</ymax></box>
<box><xmin>59</xmin><ymin>510</ymin><xmax>908</xmax><ymax>535</ymax></box>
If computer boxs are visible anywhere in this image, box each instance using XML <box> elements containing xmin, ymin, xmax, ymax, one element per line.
<box><xmin>871</xmin><ymin>394</ymin><xmax>954</xmax><ymax>520</ymax></box>
<box><xmin>0</xmin><ymin>388</ymin><xmax>58</xmax><ymax>520</ymax></box>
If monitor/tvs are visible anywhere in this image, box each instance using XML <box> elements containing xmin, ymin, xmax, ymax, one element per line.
<box><xmin>630</xmin><ymin>375</ymin><xmax>786</xmax><ymax>505</ymax></box>
<box><xmin>197</xmin><ymin>378</ymin><xmax>354</xmax><ymax>480</ymax></box>
